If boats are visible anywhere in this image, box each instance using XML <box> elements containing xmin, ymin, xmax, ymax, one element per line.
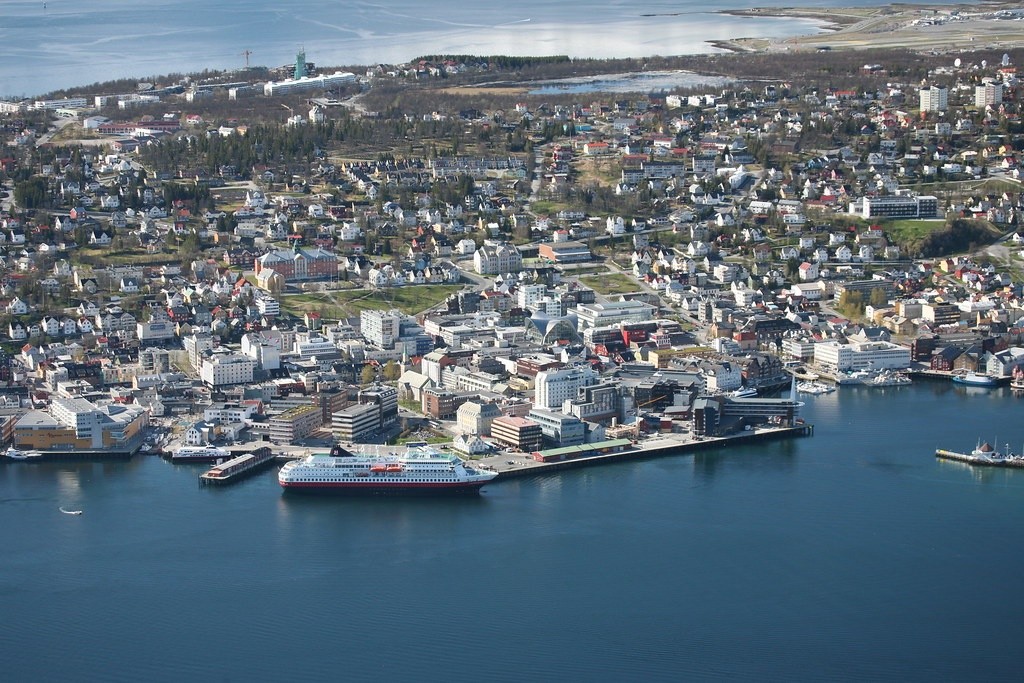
<box><xmin>276</xmin><ymin>453</ymin><xmax>499</xmax><ymax>490</ymax></box>
<box><xmin>952</xmin><ymin>373</ymin><xmax>992</xmax><ymax>383</ymax></box>
<box><xmin>835</xmin><ymin>368</ymin><xmax>912</xmax><ymax>387</ymax></box>
<box><xmin>1008</xmin><ymin>379</ymin><xmax>1023</xmax><ymax>388</ymax></box>
